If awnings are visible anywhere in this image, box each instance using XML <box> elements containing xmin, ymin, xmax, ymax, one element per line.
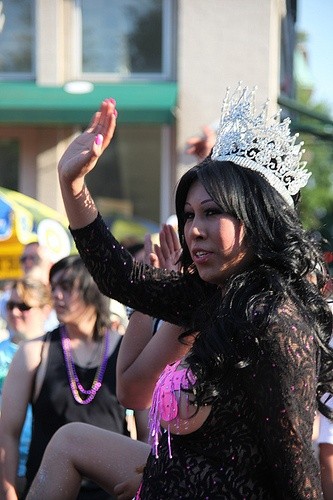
<box><xmin>0</xmin><ymin>83</ymin><xmax>177</xmax><ymax>124</ymax></box>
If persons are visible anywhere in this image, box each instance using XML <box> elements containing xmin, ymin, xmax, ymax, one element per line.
<box><xmin>25</xmin><ymin>98</ymin><xmax>333</xmax><ymax>500</ymax></box>
<box><xmin>0</xmin><ymin>124</ymin><xmax>333</xmax><ymax>500</ymax></box>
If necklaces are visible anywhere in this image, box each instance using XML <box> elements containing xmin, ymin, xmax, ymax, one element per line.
<box><xmin>68</xmin><ymin>341</ymin><xmax>102</xmax><ymax>380</ymax></box>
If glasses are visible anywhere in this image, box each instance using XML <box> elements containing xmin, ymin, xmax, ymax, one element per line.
<box><xmin>7</xmin><ymin>300</ymin><xmax>33</xmax><ymax>311</ymax></box>
<box><xmin>20</xmin><ymin>254</ymin><xmax>40</xmax><ymax>263</ymax></box>
